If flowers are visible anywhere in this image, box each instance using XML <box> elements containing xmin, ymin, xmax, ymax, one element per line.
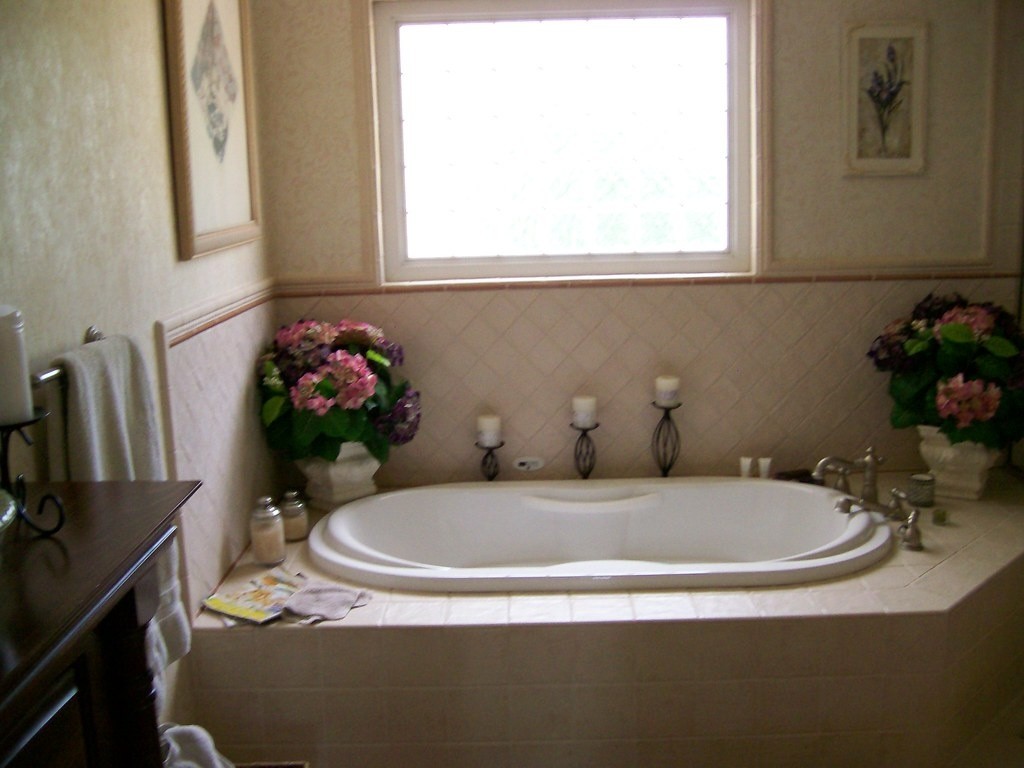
<box><xmin>256</xmin><ymin>318</ymin><xmax>419</xmax><ymax>466</ymax></box>
<box><xmin>866</xmin><ymin>291</ymin><xmax>1024</xmax><ymax>449</ymax></box>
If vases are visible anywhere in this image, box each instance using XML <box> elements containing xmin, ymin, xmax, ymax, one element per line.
<box><xmin>294</xmin><ymin>441</ymin><xmax>381</xmax><ymax>510</ymax></box>
<box><xmin>917</xmin><ymin>425</ymin><xmax>1001</xmax><ymax>500</ymax></box>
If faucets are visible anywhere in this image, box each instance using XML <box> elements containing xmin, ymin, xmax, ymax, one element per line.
<box><xmin>811</xmin><ymin>447</ymin><xmax>888</xmax><ymax>503</ymax></box>
<box><xmin>833</xmin><ymin>488</ymin><xmax>907</xmax><ymax>520</ymax></box>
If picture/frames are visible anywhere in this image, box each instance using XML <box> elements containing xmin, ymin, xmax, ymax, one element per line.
<box><xmin>164</xmin><ymin>0</ymin><xmax>263</xmax><ymax>260</ymax></box>
<box><xmin>841</xmin><ymin>21</ymin><xmax>927</xmax><ymax>175</ymax></box>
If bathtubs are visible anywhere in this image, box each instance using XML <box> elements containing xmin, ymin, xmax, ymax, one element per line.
<box><xmin>185</xmin><ymin>474</ymin><xmax>1020</xmax><ymax>767</ymax></box>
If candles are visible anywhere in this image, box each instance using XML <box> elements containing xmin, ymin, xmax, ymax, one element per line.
<box><xmin>572</xmin><ymin>397</ymin><xmax>596</xmax><ymax>428</ymax></box>
<box><xmin>477</xmin><ymin>414</ymin><xmax>501</xmax><ymax>447</ymax></box>
<box><xmin>655</xmin><ymin>375</ymin><xmax>679</xmax><ymax>407</ymax></box>
<box><xmin>0</xmin><ymin>307</ymin><xmax>34</xmax><ymax>425</ymax></box>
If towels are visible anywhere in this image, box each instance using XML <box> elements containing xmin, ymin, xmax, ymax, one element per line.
<box><xmin>281</xmin><ymin>582</ymin><xmax>375</xmax><ymax>625</ymax></box>
<box><xmin>44</xmin><ymin>334</ymin><xmax>193</xmax><ymax>727</ymax></box>
<box><xmin>160</xmin><ymin>724</ymin><xmax>234</xmax><ymax>768</ymax></box>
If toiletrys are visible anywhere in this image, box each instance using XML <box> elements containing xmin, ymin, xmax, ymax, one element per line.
<box><xmin>250</xmin><ymin>490</ymin><xmax>308</xmax><ymax>567</ymax></box>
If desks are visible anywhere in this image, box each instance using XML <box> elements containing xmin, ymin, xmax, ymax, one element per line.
<box><xmin>0</xmin><ymin>481</ymin><xmax>203</xmax><ymax>768</ymax></box>
